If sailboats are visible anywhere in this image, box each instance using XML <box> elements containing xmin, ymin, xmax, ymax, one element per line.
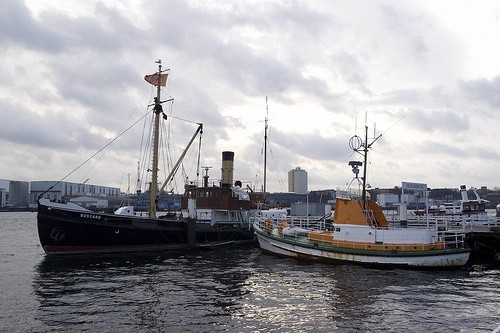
<box><xmin>37</xmin><ymin>59</ymin><xmax>253</xmax><ymax>260</ymax></box>
<box><xmin>250</xmin><ymin>96</ymin><xmax>350</xmax><ymax>210</ymax></box>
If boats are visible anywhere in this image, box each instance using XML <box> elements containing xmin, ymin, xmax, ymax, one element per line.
<box><xmin>414</xmin><ymin>185</ymin><xmax>490</xmax><ymax>215</ymax></box>
<box><xmin>253</xmin><ymin>110</ymin><xmax>472</xmax><ymax>269</ymax></box>
<box><xmin>118</xmin><ymin>162</ymin><xmax>183</xmax><ymax>212</ymax></box>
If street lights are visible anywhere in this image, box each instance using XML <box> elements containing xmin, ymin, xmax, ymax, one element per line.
<box><xmin>305</xmin><ymin>191</ymin><xmax>310</xmax><ymax>216</ymax></box>
